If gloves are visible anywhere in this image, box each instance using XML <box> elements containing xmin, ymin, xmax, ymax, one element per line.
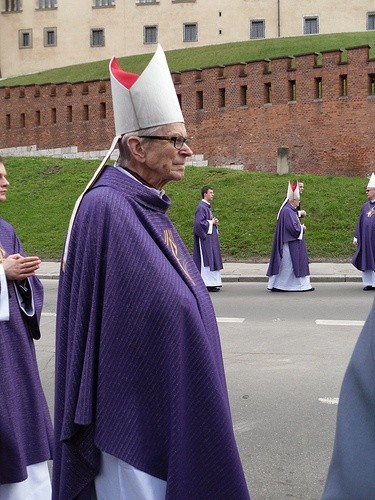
<box><xmin>352</xmin><ymin>237</ymin><xmax>359</xmax><ymax>247</ymax></box>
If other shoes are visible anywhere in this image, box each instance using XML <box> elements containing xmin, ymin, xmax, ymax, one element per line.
<box><xmin>363</xmin><ymin>286</ymin><xmax>375</xmax><ymax>290</ymax></box>
<box><xmin>207</xmin><ymin>287</ymin><xmax>221</xmax><ymax>291</ymax></box>
<box><xmin>306</xmin><ymin>288</ymin><xmax>315</xmax><ymax>291</ymax></box>
<box><xmin>271</xmin><ymin>288</ymin><xmax>279</xmax><ymax>292</ymax></box>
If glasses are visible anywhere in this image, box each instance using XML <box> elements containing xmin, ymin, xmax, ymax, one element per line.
<box><xmin>138</xmin><ymin>135</ymin><xmax>193</xmax><ymax>150</ymax></box>
<box><xmin>364</xmin><ymin>188</ymin><xmax>374</xmax><ymax>192</ymax></box>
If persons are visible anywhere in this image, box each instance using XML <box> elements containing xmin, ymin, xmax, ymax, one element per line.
<box><xmin>52</xmin><ymin>42</ymin><xmax>251</xmax><ymax>500</ymax></box>
<box><xmin>350</xmin><ymin>171</ymin><xmax>375</xmax><ymax>291</ymax></box>
<box><xmin>295</xmin><ymin>180</ymin><xmax>307</xmax><ymax>219</ymax></box>
<box><xmin>192</xmin><ymin>186</ymin><xmax>224</xmax><ymax>292</ymax></box>
<box><xmin>0</xmin><ymin>155</ymin><xmax>55</xmax><ymax>500</ymax></box>
<box><xmin>265</xmin><ymin>179</ymin><xmax>315</xmax><ymax>292</ymax></box>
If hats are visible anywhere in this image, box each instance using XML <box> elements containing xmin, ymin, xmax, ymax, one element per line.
<box><xmin>62</xmin><ymin>41</ymin><xmax>186</xmax><ymax>274</ymax></box>
<box><xmin>367</xmin><ymin>172</ymin><xmax>375</xmax><ymax>188</ymax></box>
<box><xmin>276</xmin><ymin>179</ymin><xmax>300</xmax><ymax>220</ymax></box>
